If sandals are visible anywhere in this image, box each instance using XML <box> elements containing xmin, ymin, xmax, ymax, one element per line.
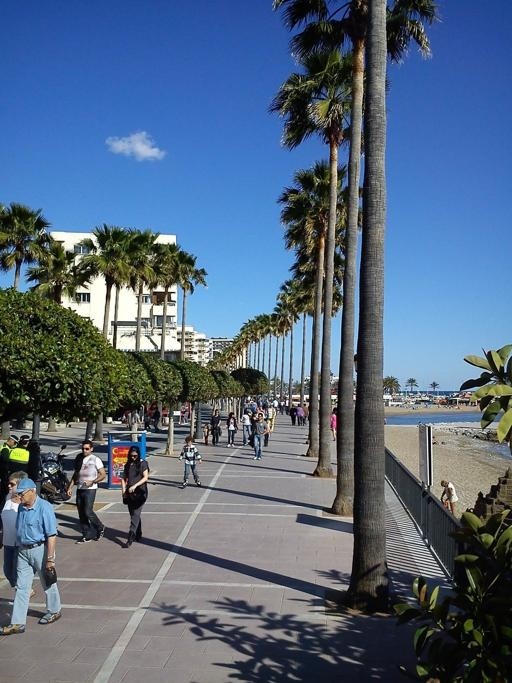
<box><xmin>0</xmin><ymin>623</ymin><xmax>25</xmax><ymax>635</ymax></box>
<box><xmin>39</xmin><ymin>611</ymin><xmax>61</xmax><ymax>625</ymax></box>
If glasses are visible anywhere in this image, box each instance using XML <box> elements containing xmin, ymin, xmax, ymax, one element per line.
<box><xmin>6</xmin><ymin>482</ymin><xmax>16</xmax><ymax>487</ymax></box>
<box><xmin>82</xmin><ymin>447</ymin><xmax>90</xmax><ymax>450</ymax></box>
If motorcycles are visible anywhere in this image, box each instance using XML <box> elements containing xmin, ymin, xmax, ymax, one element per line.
<box><xmin>40</xmin><ymin>441</ymin><xmax>73</xmax><ymax>502</ymax></box>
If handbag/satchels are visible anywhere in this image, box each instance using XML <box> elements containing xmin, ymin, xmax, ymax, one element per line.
<box><xmin>45</xmin><ymin>567</ymin><xmax>57</xmax><ymax>586</ymax></box>
<box><xmin>123</xmin><ymin>489</ymin><xmax>140</xmax><ymax>505</ymax></box>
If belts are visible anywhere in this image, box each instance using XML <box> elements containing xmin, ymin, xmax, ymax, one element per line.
<box><xmin>23</xmin><ymin>539</ymin><xmax>47</xmax><ymax>549</ymax></box>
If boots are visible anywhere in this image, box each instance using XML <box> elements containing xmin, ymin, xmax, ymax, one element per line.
<box><xmin>125</xmin><ymin>531</ymin><xmax>136</xmax><ymax>548</ymax></box>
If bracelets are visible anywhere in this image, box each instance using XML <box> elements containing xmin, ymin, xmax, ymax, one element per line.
<box><xmin>47</xmin><ymin>555</ymin><xmax>54</xmax><ymax>559</ymax></box>
<box><xmin>46</xmin><ymin>560</ymin><xmax>55</xmax><ymax>564</ymax></box>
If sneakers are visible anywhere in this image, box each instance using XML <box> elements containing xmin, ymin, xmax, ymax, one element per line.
<box><xmin>197</xmin><ymin>480</ymin><xmax>201</xmax><ymax>487</ymax></box>
<box><xmin>95</xmin><ymin>527</ymin><xmax>105</xmax><ymax>541</ymax></box>
<box><xmin>76</xmin><ymin>537</ymin><xmax>91</xmax><ymax>543</ymax></box>
<box><xmin>183</xmin><ymin>479</ymin><xmax>189</xmax><ymax>487</ymax></box>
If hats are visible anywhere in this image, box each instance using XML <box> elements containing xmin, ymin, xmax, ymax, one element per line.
<box><xmin>15</xmin><ymin>478</ymin><xmax>36</xmax><ymax>494</ymax></box>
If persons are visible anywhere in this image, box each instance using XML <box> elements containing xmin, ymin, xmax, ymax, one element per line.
<box><xmin>330</xmin><ymin>411</ymin><xmax>337</xmax><ymax>441</ymax></box>
<box><xmin>235</xmin><ymin>397</ymin><xmax>309</xmax><ymax>460</ymax></box>
<box><xmin>143</xmin><ymin>415</ymin><xmax>151</xmax><ymax>430</ymax></box>
<box><xmin>225</xmin><ymin>412</ymin><xmax>238</xmax><ymax>447</ymax></box>
<box><xmin>202</xmin><ymin>425</ymin><xmax>211</xmax><ymax>446</ymax></box>
<box><xmin>0</xmin><ymin>434</ymin><xmax>31</xmax><ymax>502</ymax></box>
<box><xmin>210</xmin><ymin>409</ymin><xmax>221</xmax><ymax>446</ymax></box>
<box><xmin>176</xmin><ymin>436</ymin><xmax>202</xmax><ymax>489</ymax></box>
<box><xmin>0</xmin><ymin>471</ymin><xmax>36</xmax><ymax>599</ymax></box>
<box><xmin>67</xmin><ymin>440</ymin><xmax>106</xmax><ymax>544</ymax></box>
<box><xmin>150</xmin><ymin>407</ymin><xmax>161</xmax><ymax>433</ymax></box>
<box><xmin>120</xmin><ymin>445</ymin><xmax>150</xmax><ymax>547</ymax></box>
<box><xmin>440</xmin><ymin>480</ymin><xmax>458</xmax><ymax>516</ymax></box>
<box><xmin>384</xmin><ymin>417</ymin><xmax>387</xmax><ymax>425</ymax></box>
<box><xmin>0</xmin><ymin>478</ymin><xmax>63</xmax><ymax>636</ymax></box>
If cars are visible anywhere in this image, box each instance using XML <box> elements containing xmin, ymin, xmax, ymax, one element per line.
<box><xmin>243</xmin><ymin>402</ymin><xmax>257</xmax><ymax>414</ymax></box>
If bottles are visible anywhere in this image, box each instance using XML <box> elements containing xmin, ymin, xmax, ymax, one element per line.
<box><xmin>259</xmin><ymin>430</ymin><xmax>263</xmax><ymax>434</ymax></box>
<box><xmin>121</xmin><ymin>479</ymin><xmax>126</xmax><ymax>493</ymax></box>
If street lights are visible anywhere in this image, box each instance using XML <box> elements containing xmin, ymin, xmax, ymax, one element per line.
<box><xmin>354</xmin><ymin>353</ymin><xmax>359</xmax><ymax>373</ymax></box>
<box><xmin>330</xmin><ymin>369</ymin><xmax>335</xmax><ymax>408</ymax></box>
